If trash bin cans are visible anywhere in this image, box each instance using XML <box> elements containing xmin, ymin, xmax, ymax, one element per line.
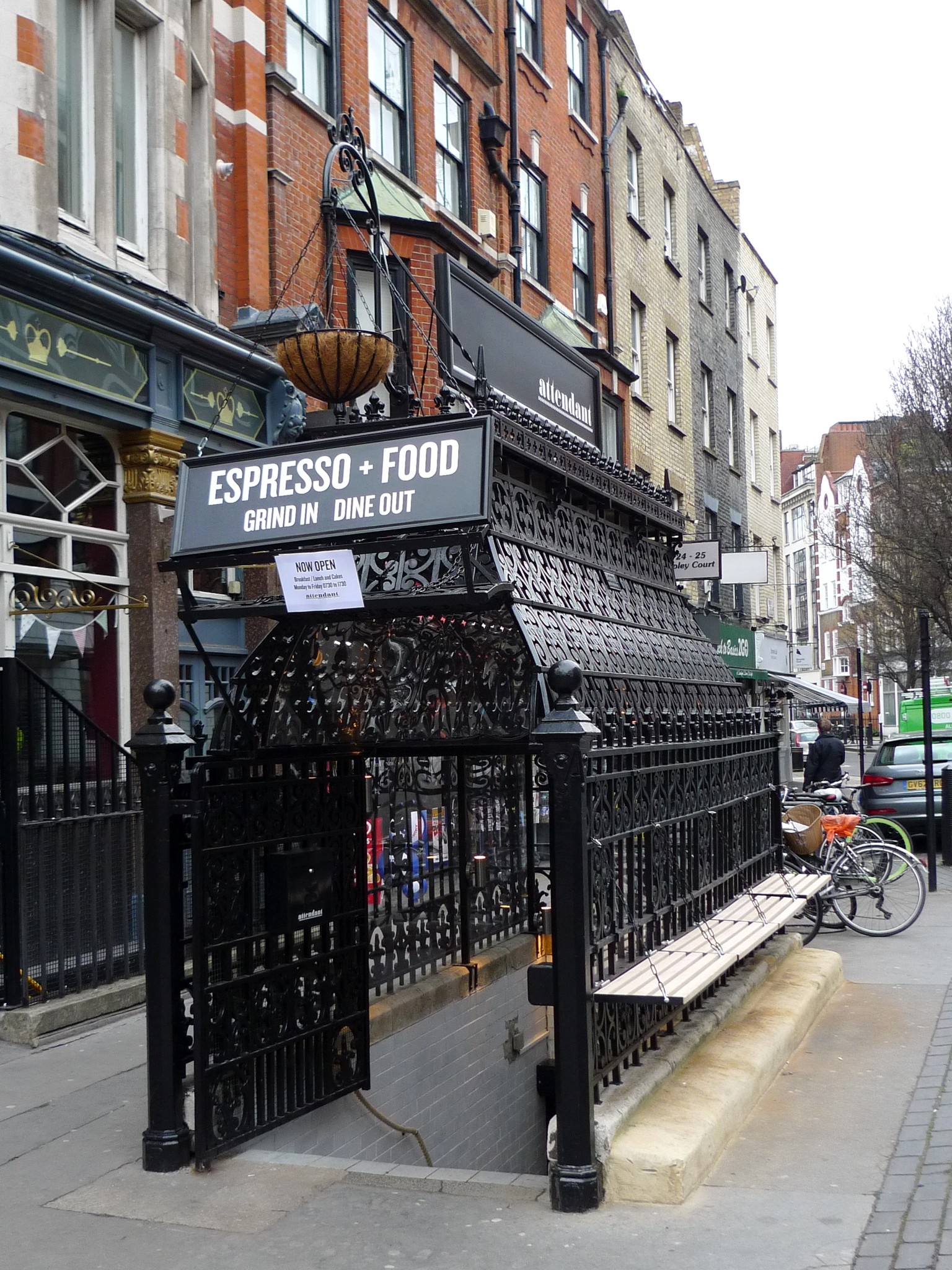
<box><xmin>792</xmin><ymin>747</ymin><xmax>803</xmax><ymax>772</ymax></box>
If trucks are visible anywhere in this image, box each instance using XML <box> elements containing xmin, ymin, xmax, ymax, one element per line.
<box><xmin>899</xmin><ymin>669</ymin><xmax>952</xmax><ymax>744</ymax></box>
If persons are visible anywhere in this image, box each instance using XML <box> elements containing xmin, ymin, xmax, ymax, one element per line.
<box><xmin>803</xmin><ymin>718</ymin><xmax>846</xmax><ymax>793</ymax></box>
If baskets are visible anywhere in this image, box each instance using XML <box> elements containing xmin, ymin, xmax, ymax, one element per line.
<box><xmin>782</xmin><ymin>804</ymin><xmax>823</xmax><ymax>855</ymax></box>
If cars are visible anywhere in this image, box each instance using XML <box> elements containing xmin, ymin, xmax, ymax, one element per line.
<box><xmin>790</xmin><ymin>720</ymin><xmax>819</xmax><ymax>741</ymax></box>
<box><xmin>856</xmin><ymin>730</ymin><xmax>952</xmax><ymax>836</ymax></box>
<box><xmin>789</xmin><ymin>727</ymin><xmax>820</xmax><ymax>762</ymax></box>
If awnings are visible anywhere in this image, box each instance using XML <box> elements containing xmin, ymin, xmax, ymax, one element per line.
<box><xmin>772</xmin><ymin>674</ymin><xmax>872</xmax><ymax>714</ymax></box>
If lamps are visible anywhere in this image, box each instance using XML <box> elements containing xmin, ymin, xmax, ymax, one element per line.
<box><xmin>217</xmin><ymin>160</ymin><xmax>234</xmax><ymax>181</ymax></box>
<box><xmin>758</xmin><ymin>623</ymin><xmax>788</xmax><ymax>630</ymax></box>
<box><xmin>756</xmin><ymin>688</ymin><xmax>795</xmax><ymax>703</ymax></box>
<box><xmin>534</xmin><ymin>906</ymin><xmax>552</xmax><ymax>936</ymax></box>
<box><xmin>466</xmin><ymin>855</ymin><xmax>487</xmax><ymax>892</ymax></box>
<box><xmin>778</xmin><ymin>631</ymin><xmax>802</xmax><ymax>637</ymax></box>
<box><xmin>693</xmin><ymin>609</ymin><xmax>711</xmax><ymax>617</ymax></box>
<box><xmin>714</xmin><ymin>606</ymin><xmax>747</xmax><ymax>616</ymax></box>
<box><xmin>739</xmin><ymin>616</ymin><xmax>771</xmax><ymax>623</ymax></box>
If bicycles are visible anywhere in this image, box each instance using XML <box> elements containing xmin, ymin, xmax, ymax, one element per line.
<box><xmin>777</xmin><ymin>771</ymin><xmax>930</xmax><ymax>947</ymax></box>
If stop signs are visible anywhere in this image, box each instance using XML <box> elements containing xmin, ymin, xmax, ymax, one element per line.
<box><xmin>840</xmin><ymin>685</ymin><xmax>845</xmax><ymax>694</ymax></box>
<box><xmin>862</xmin><ymin>682</ymin><xmax>868</xmax><ymax>693</ymax></box>
